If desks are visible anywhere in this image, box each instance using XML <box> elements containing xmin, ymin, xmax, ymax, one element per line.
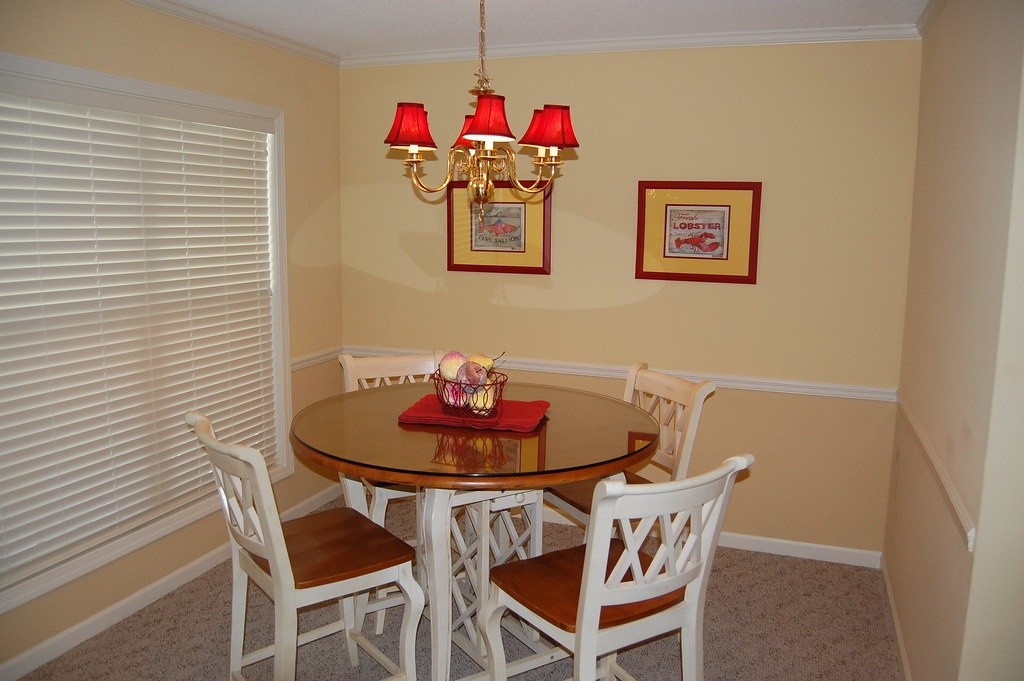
<box><xmin>289</xmin><ymin>383</ymin><xmax>660</xmax><ymax>681</ymax></box>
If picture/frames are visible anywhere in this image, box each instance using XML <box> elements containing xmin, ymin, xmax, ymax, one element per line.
<box><xmin>634</xmin><ymin>181</ymin><xmax>762</xmax><ymax>284</ymax></box>
<box><xmin>446</xmin><ymin>180</ymin><xmax>552</xmax><ymax>274</ymax></box>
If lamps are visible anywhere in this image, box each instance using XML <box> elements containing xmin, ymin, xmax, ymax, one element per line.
<box><xmin>383</xmin><ymin>0</ymin><xmax>579</xmax><ymax>223</ymax></box>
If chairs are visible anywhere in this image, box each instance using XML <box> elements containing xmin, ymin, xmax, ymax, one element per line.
<box><xmin>341</xmin><ymin>349</ymin><xmax>443</xmax><ymax>528</ymax></box>
<box><xmin>184</xmin><ymin>412</ymin><xmax>425</xmax><ymax>681</ymax></box>
<box><xmin>479</xmin><ymin>453</ymin><xmax>756</xmax><ymax>681</ymax></box>
<box><xmin>544</xmin><ymin>362</ymin><xmax>715</xmax><ymax>545</ymax></box>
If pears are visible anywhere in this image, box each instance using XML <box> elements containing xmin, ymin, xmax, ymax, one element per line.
<box><xmin>468</xmin><ymin>351</ymin><xmax>505</xmax><ymax>372</ymax></box>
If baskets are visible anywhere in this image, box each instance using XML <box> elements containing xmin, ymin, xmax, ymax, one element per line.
<box><xmin>429</xmin><ymin>369</ymin><xmax>508</xmax><ymax>418</ymax></box>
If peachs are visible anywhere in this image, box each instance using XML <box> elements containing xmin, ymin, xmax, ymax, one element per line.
<box><xmin>440</xmin><ymin>350</ymin><xmax>494</xmax><ymax>411</ymax></box>
